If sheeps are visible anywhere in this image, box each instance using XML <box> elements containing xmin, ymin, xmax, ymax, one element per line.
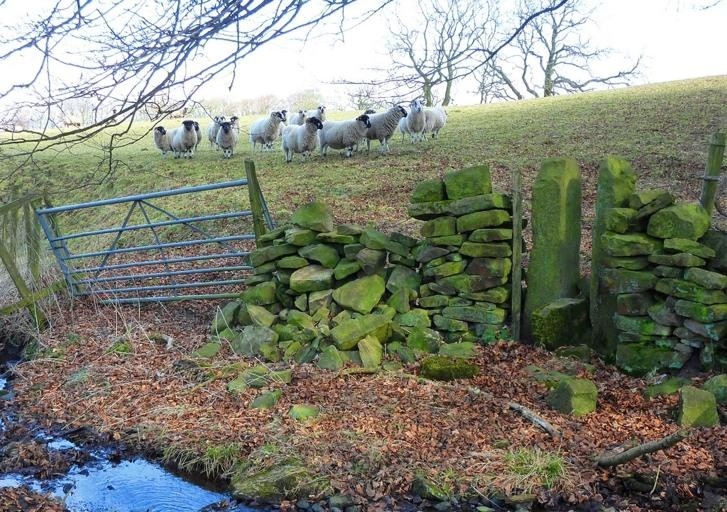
<box><xmin>152</xmin><ymin>97</ymin><xmax>446</xmax><ymax>164</ymax></box>
